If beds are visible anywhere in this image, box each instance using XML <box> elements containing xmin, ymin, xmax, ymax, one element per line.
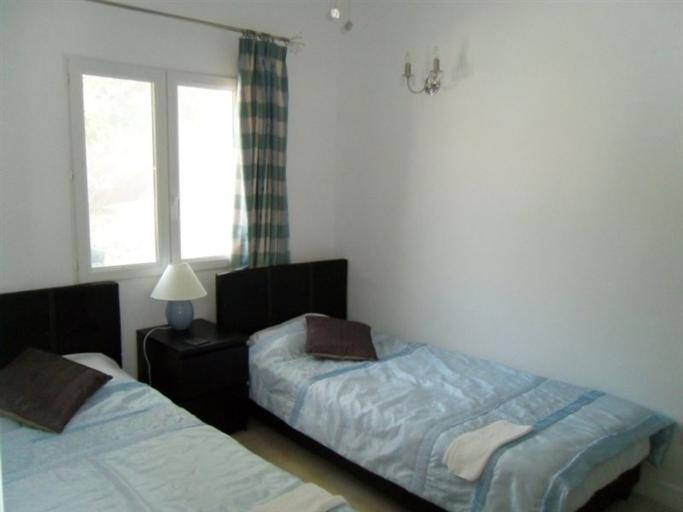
<box><xmin>214</xmin><ymin>259</ymin><xmax>679</xmax><ymax>512</ymax></box>
<box><xmin>0</xmin><ymin>281</ymin><xmax>350</xmax><ymax>512</ymax></box>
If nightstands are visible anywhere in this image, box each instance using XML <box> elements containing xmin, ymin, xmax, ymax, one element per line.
<box><xmin>136</xmin><ymin>318</ymin><xmax>251</xmax><ymax>438</ymax></box>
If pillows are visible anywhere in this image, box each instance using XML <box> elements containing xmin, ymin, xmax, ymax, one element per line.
<box><xmin>0</xmin><ymin>347</ymin><xmax>112</xmax><ymax>435</ymax></box>
<box><xmin>246</xmin><ymin>304</ymin><xmax>379</xmax><ymax>363</ymax></box>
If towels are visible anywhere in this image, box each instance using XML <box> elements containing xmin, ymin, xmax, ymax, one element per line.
<box><xmin>250</xmin><ymin>481</ymin><xmax>346</xmax><ymax>512</ymax></box>
<box><xmin>441</xmin><ymin>420</ymin><xmax>533</xmax><ymax>482</ymax></box>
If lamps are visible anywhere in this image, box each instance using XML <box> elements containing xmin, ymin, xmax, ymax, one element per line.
<box><xmin>404</xmin><ymin>57</ymin><xmax>443</xmax><ymax>98</ymax></box>
<box><xmin>149</xmin><ymin>262</ymin><xmax>207</xmax><ymax>333</ymax></box>
